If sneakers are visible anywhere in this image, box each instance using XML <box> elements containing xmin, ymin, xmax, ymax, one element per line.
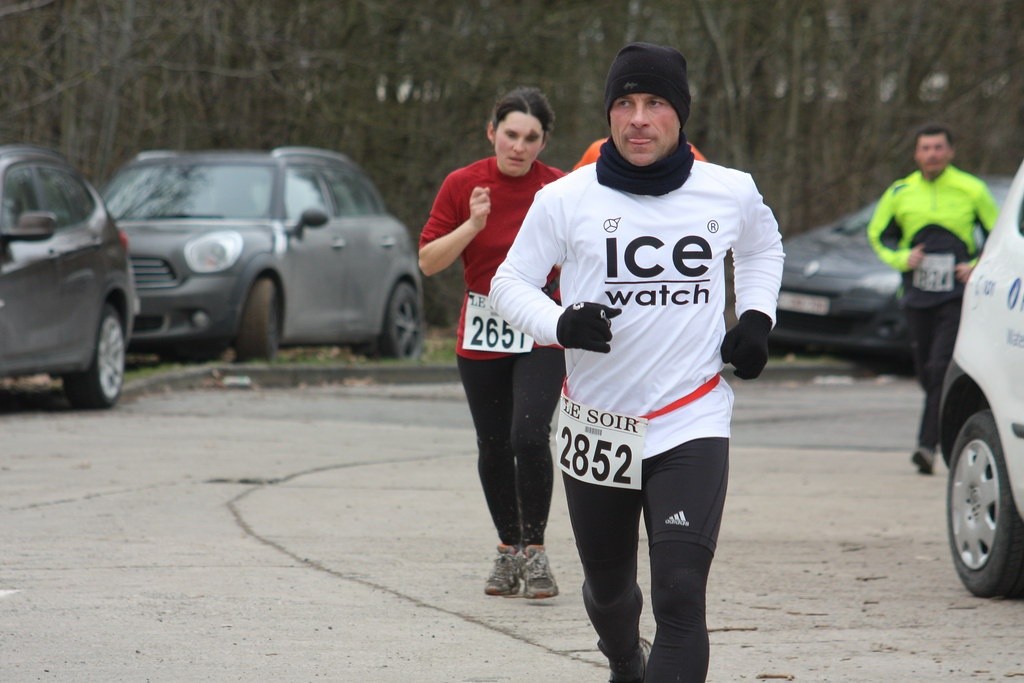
<box><xmin>485</xmin><ymin>544</ymin><xmax>521</xmax><ymax>595</ymax></box>
<box><xmin>516</xmin><ymin>545</ymin><xmax>559</xmax><ymax>599</ymax></box>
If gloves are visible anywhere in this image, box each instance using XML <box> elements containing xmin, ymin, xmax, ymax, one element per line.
<box><xmin>556</xmin><ymin>301</ymin><xmax>622</xmax><ymax>353</ymax></box>
<box><xmin>721</xmin><ymin>309</ymin><xmax>772</xmax><ymax>379</ymax></box>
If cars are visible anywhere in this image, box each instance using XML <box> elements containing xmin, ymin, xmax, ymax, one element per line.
<box><xmin>768</xmin><ymin>176</ymin><xmax>1012</xmax><ymax>374</ymax></box>
<box><xmin>937</xmin><ymin>152</ymin><xmax>1024</xmax><ymax>598</ymax></box>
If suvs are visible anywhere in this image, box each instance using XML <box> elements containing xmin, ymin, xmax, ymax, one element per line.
<box><xmin>101</xmin><ymin>146</ymin><xmax>425</xmax><ymax>364</ymax></box>
<box><xmin>0</xmin><ymin>146</ymin><xmax>142</xmax><ymax>405</ymax></box>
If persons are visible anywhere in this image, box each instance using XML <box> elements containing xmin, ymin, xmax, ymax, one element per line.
<box><xmin>419</xmin><ymin>89</ymin><xmax>567</xmax><ymax>598</ymax></box>
<box><xmin>489</xmin><ymin>42</ymin><xmax>785</xmax><ymax>683</ymax></box>
<box><xmin>867</xmin><ymin>125</ymin><xmax>998</xmax><ymax>476</ymax></box>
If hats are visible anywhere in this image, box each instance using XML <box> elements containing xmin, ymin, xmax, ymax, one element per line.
<box><xmin>604</xmin><ymin>42</ymin><xmax>691</xmax><ymax>129</ymax></box>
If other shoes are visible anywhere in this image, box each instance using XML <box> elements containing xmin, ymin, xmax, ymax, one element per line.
<box><xmin>912</xmin><ymin>447</ymin><xmax>935</xmax><ymax>475</ymax></box>
<box><xmin>597</xmin><ymin>637</ymin><xmax>653</xmax><ymax>683</ymax></box>
